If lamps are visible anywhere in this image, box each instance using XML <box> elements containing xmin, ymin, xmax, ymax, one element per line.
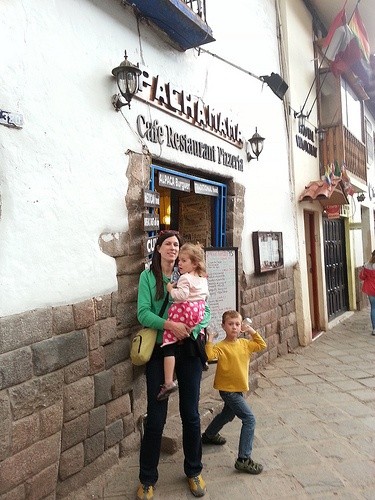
<box><xmin>357</xmin><ymin>192</ymin><xmax>367</xmax><ymax>202</ymax></box>
<box><xmin>260</xmin><ymin>72</ymin><xmax>289</xmax><ymax>101</ymax></box>
<box><xmin>247</xmin><ymin>127</ymin><xmax>265</xmax><ymax>161</ymax></box>
<box><xmin>112</xmin><ymin>50</ymin><xmax>142</xmax><ymax>111</ymax></box>
<box><xmin>315</xmin><ymin>126</ymin><xmax>326</xmax><ymax>141</ymax></box>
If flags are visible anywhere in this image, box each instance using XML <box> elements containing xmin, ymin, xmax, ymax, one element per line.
<box><xmin>322</xmin><ymin>3</ymin><xmax>370</xmax><ymax>80</ymax></box>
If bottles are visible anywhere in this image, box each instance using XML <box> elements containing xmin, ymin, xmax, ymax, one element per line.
<box><xmin>169</xmin><ymin>267</ymin><xmax>181</xmax><ymax>303</ymax></box>
<box><xmin>238</xmin><ymin>318</ymin><xmax>252</xmax><ymax>337</ymax></box>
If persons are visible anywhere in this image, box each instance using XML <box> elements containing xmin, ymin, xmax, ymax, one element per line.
<box><xmin>358</xmin><ymin>249</ymin><xmax>375</xmax><ymax>335</ymax></box>
<box><xmin>156</xmin><ymin>241</ymin><xmax>210</xmax><ymax>402</ymax></box>
<box><xmin>204</xmin><ymin>310</ymin><xmax>267</xmax><ymax>475</ymax></box>
<box><xmin>135</xmin><ymin>231</ymin><xmax>212</xmax><ymax>499</ymax></box>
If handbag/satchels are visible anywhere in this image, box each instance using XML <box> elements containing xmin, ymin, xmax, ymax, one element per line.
<box><xmin>130</xmin><ymin>327</ymin><xmax>157</xmax><ymax>367</ymax></box>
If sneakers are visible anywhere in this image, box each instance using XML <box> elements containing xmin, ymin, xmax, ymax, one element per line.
<box><xmin>137</xmin><ymin>480</ymin><xmax>154</xmax><ymax>500</ymax></box>
<box><xmin>202</xmin><ymin>433</ymin><xmax>227</xmax><ymax>445</ymax></box>
<box><xmin>235</xmin><ymin>457</ymin><xmax>263</xmax><ymax>474</ymax></box>
<box><xmin>188</xmin><ymin>474</ymin><xmax>207</xmax><ymax>498</ymax></box>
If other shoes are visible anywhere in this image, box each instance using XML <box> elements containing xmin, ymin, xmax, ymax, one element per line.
<box><xmin>372</xmin><ymin>331</ymin><xmax>375</xmax><ymax>335</ymax></box>
<box><xmin>157</xmin><ymin>382</ymin><xmax>178</xmax><ymax>401</ymax></box>
<box><xmin>203</xmin><ymin>361</ymin><xmax>210</xmax><ymax>371</ymax></box>
